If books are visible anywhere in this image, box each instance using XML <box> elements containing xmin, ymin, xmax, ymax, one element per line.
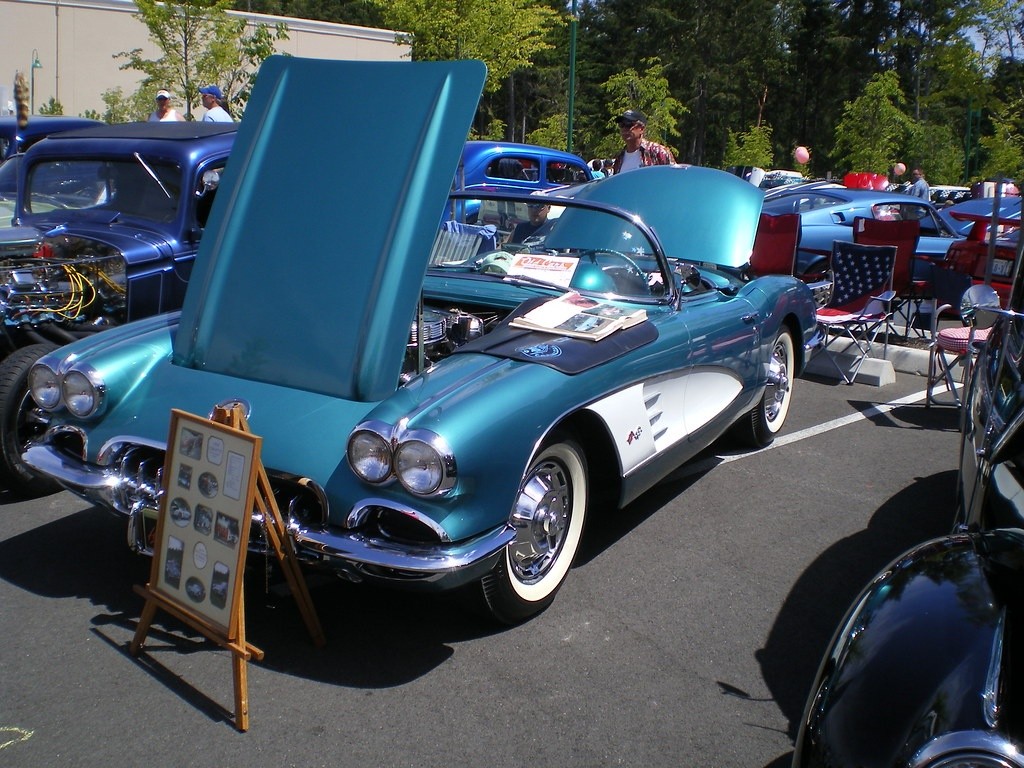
<box><xmin>507</xmin><ymin>289</ymin><xmax>649</xmax><ymax>340</ymax></box>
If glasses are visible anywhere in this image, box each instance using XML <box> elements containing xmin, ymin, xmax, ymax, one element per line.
<box><xmin>526</xmin><ymin>204</ymin><xmax>543</xmax><ymax>208</ymax></box>
<box><xmin>617</xmin><ymin>122</ymin><xmax>642</xmax><ymax>128</ymax></box>
<box><xmin>157</xmin><ymin>97</ymin><xmax>167</xmax><ymax>101</ymax></box>
<box><xmin>913</xmin><ymin>173</ymin><xmax>920</xmax><ymax>175</ymax></box>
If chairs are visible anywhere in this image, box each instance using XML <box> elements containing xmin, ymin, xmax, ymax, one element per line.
<box><xmin>749</xmin><ymin>213</ymin><xmax>830</xmax><ymax>283</ymax></box>
<box><xmin>925</xmin><ymin>298</ymin><xmax>994</xmax><ymax>410</ymax></box>
<box><xmin>806</xmin><ymin>215</ymin><xmax>973</xmax><ymax>386</ymax></box>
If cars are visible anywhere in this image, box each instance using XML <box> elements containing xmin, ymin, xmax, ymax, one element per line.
<box><xmin>0</xmin><ymin>122</ymin><xmax>243</xmax><ymax>496</ymax></box>
<box><xmin>21</xmin><ymin>54</ymin><xmax>833</xmax><ymax>624</ymax></box>
<box><xmin>937</xmin><ymin>210</ymin><xmax>1024</xmax><ymax>315</ymax></box>
<box><xmin>438</xmin><ymin>140</ymin><xmax>595</xmax><ymax>232</ymax></box>
<box><xmin>761</xmin><ymin>188</ymin><xmax>967</xmax><ymax>281</ymax></box>
<box><xmin>791</xmin><ymin>246</ymin><xmax>1024</xmax><ymax>768</ymax></box>
<box><xmin>0</xmin><ymin>116</ymin><xmax>112</xmax><ymax>194</ymax></box>
<box><xmin>917</xmin><ymin>196</ymin><xmax>1024</xmax><ymax>244</ymax></box>
<box><xmin>724</xmin><ymin>164</ymin><xmax>847</xmax><ymax>206</ymax></box>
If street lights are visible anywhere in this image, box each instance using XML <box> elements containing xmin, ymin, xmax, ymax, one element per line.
<box><xmin>31</xmin><ymin>49</ymin><xmax>42</xmax><ymax>114</ymax></box>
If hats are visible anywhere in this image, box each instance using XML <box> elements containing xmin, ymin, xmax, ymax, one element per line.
<box><xmin>524</xmin><ymin>190</ymin><xmax>550</xmax><ymax>204</ymax></box>
<box><xmin>613</xmin><ymin>110</ymin><xmax>647</xmax><ymax>125</ymax></box>
<box><xmin>198</xmin><ymin>85</ymin><xmax>222</xmax><ymax>100</ymax></box>
<box><xmin>156</xmin><ymin>90</ymin><xmax>170</xmax><ymax>99</ymax></box>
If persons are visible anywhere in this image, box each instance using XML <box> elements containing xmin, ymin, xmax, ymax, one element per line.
<box><xmin>602</xmin><ymin>160</ymin><xmax>614</xmax><ymax>177</ymax></box>
<box><xmin>894</xmin><ymin>163</ymin><xmax>930</xmax><ymax>219</ymax></box>
<box><xmin>511</xmin><ymin>202</ymin><xmax>556</xmax><ymax>249</ymax></box>
<box><xmin>589</xmin><ymin>160</ymin><xmax>605</xmax><ymax>178</ymax></box>
<box><xmin>148</xmin><ymin>88</ymin><xmax>187</xmax><ymax>122</ymax></box>
<box><xmin>936</xmin><ymin>200</ymin><xmax>955</xmax><ymax>237</ymax></box>
<box><xmin>197</xmin><ymin>86</ymin><xmax>233</xmax><ymax>122</ymax></box>
<box><xmin>613</xmin><ymin>109</ymin><xmax>676</xmax><ymax>176</ymax></box>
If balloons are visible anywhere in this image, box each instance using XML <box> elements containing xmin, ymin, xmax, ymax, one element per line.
<box><xmin>894</xmin><ymin>163</ymin><xmax>907</xmax><ymax>176</ymax></box>
<box><xmin>796</xmin><ymin>146</ymin><xmax>809</xmax><ymax>163</ymax></box>
